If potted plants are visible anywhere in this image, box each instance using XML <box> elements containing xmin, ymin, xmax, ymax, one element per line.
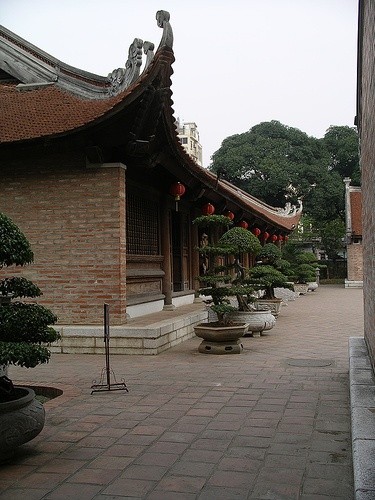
<box><xmin>219</xmin><ymin>227</ymin><xmax>276</xmax><ymax>337</ymax></box>
<box><xmin>193</xmin><ymin>215</ymin><xmax>255</xmax><ymax>354</ymax></box>
<box><xmin>0</xmin><ymin>210</ymin><xmax>62</xmax><ymax>451</ymax></box>
<box><xmin>290</xmin><ymin>254</ymin><xmax>327</xmax><ymax>295</ymax></box>
<box><xmin>248</xmin><ymin>242</ymin><xmax>291</xmax><ymax>317</ymax></box>
